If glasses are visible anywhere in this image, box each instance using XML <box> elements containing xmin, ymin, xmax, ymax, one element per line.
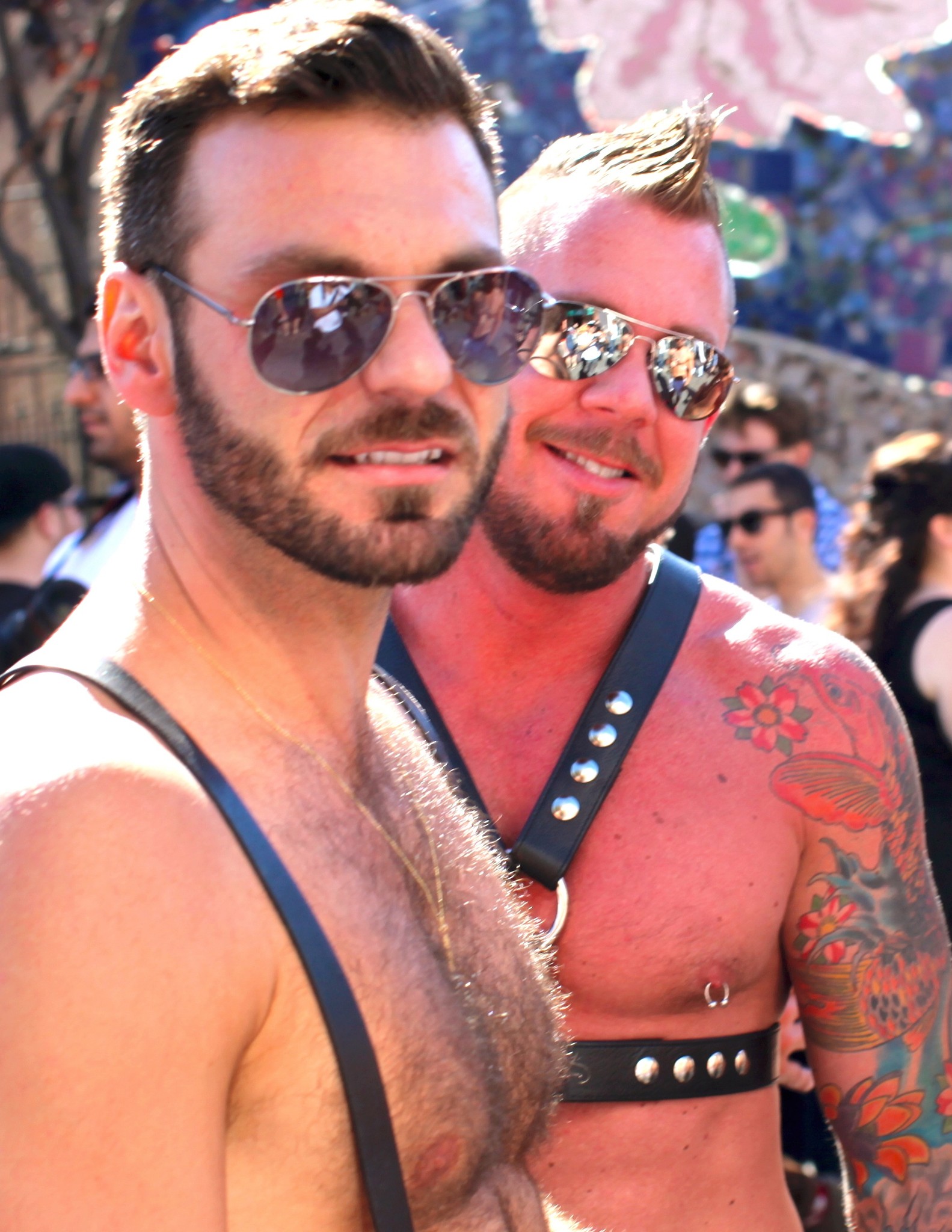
<box><xmin>710</xmin><ymin>448</ymin><xmax>763</xmax><ymax>468</ymax></box>
<box><xmin>527</xmin><ymin>292</ymin><xmax>740</xmax><ymax>421</ymax></box>
<box><xmin>157</xmin><ymin>265</ymin><xmax>548</xmax><ymax>393</ymax></box>
<box><xmin>721</xmin><ymin>507</ymin><xmax>795</xmax><ymax>536</ymax></box>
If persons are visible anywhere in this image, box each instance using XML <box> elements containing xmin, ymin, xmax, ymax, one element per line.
<box><xmin>269</xmin><ymin>279</ymin><xmax>366</xmax><ymax>375</ymax></box>
<box><xmin>712</xmin><ymin>429</ymin><xmax>952</xmax><ymax>1232</ymax></box>
<box><xmin>0</xmin><ymin>0</ymin><xmax>584</xmax><ymax>1232</ymax></box>
<box><xmin>688</xmin><ymin>381</ymin><xmax>852</xmax><ymax>614</ymax></box>
<box><xmin>650</xmin><ymin>334</ymin><xmax>731</xmax><ymax>419</ymax></box>
<box><xmin>0</xmin><ymin>298</ymin><xmax>145</xmax><ymax>679</ymax></box>
<box><xmin>548</xmin><ymin>304</ymin><xmax>613</xmax><ymax>378</ymax></box>
<box><xmin>381</xmin><ymin>97</ymin><xmax>952</xmax><ymax>1232</ymax></box>
<box><xmin>441</xmin><ymin>269</ymin><xmax>538</xmax><ymax>378</ymax></box>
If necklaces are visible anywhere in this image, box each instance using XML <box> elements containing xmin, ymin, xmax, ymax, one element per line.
<box><xmin>110</xmin><ymin>561</ymin><xmax>466</xmax><ymax>989</ymax></box>
